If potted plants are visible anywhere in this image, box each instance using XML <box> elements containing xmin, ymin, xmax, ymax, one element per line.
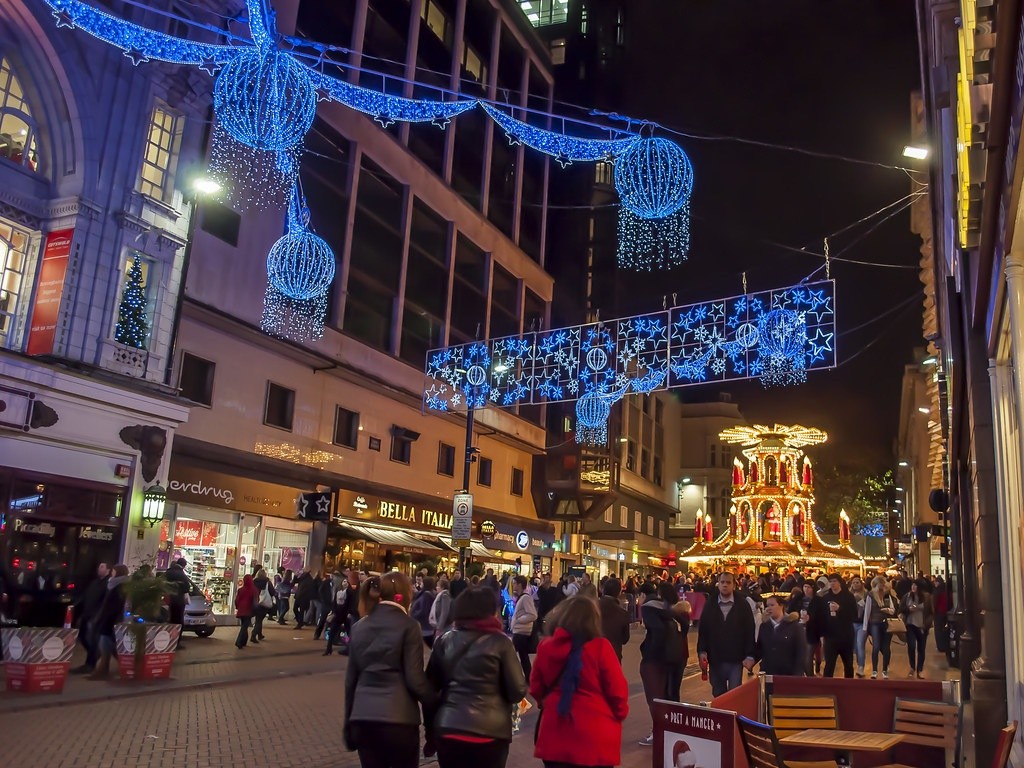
<box><xmin>111</xmin><ymin>567</ymin><xmax>188</xmax><ymax>682</ymax></box>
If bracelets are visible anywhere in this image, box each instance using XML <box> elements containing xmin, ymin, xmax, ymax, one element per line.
<box><xmin>3</xmin><ymin>593</ymin><xmax>7</xmax><ymax>596</ymax></box>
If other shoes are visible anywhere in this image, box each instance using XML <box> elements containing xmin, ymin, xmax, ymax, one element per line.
<box><xmin>856</xmin><ymin>665</ymin><xmax>864</xmax><ymax>676</ymax></box>
<box><xmin>251</xmin><ymin>636</ymin><xmax>258</xmax><ymax>643</ymax></box>
<box><xmin>908</xmin><ymin>671</ymin><xmax>913</xmax><ymax>677</ymax></box>
<box><xmin>322</xmin><ymin>640</ymin><xmax>332</xmax><ymax>655</ymax></box>
<box><xmin>638</xmin><ymin>733</ymin><xmax>653</xmax><ymax>745</ymax></box>
<box><xmin>258</xmin><ymin>633</ymin><xmax>264</xmax><ymax>640</ymax></box>
<box><xmin>882</xmin><ymin>671</ymin><xmax>888</xmax><ymax>678</ymax></box>
<box><xmin>917</xmin><ymin>672</ymin><xmax>925</xmax><ymax>679</ymax></box>
<box><xmin>871</xmin><ymin>671</ymin><xmax>879</xmax><ymax>678</ymax></box>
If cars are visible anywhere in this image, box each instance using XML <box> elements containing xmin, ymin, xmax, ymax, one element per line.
<box><xmin>155</xmin><ymin>566</ymin><xmax>216</xmax><ymax>638</ymax></box>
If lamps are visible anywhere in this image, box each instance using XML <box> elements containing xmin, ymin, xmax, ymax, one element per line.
<box><xmin>900</xmin><ymin>140</ymin><xmax>940</xmax><ymax>167</ymax></box>
<box><xmin>899</xmin><ymin>460</ymin><xmax>912</xmax><ymax>469</ymax></box>
<box><xmin>140</xmin><ymin>479</ymin><xmax>169</xmax><ymax>530</ymax></box>
<box><xmin>918</xmin><ymin>405</ymin><xmax>931</xmax><ymax>414</ymax></box>
<box><xmin>922</xmin><ymin>355</ymin><xmax>941</xmax><ymax>370</ymax></box>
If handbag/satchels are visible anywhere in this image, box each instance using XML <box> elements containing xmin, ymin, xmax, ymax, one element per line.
<box><xmin>259</xmin><ymin>582</ymin><xmax>273</xmax><ymax>608</ymax></box>
<box><xmin>886</xmin><ymin>608</ymin><xmax>907</xmax><ymax>632</ymax></box>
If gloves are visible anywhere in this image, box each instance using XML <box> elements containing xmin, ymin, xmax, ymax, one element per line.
<box><xmin>342</xmin><ymin>719</ymin><xmax>362</xmax><ymax>752</ymax></box>
<box><xmin>424</xmin><ymin>727</ymin><xmax>437</xmax><ymax>758</ymax></box>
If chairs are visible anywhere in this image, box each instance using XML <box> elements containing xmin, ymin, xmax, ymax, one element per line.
<box><xmin>767</xmin><ymin>693</ymin><xmax>840</xmax><ymax>735</ymax></box>
<box><xmin>737</xmin><ymin>715</ymin><xmax>782</xmax><ymax>768</ymax></box>
<box><xmin>990</xmin><ymin>719</ymin><xmax>1018</xmax><ymax>768</ymax></box>
<box><xmin>892</xmin><ymin>697</ymin><xmax>965</xmax><ymax>768</ymax></box>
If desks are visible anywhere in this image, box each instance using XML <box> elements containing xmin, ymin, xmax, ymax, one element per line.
<box><xmin>776</xmin><ymin>725</ymin><xmax>905</xmax><ymax>768</ymax></box>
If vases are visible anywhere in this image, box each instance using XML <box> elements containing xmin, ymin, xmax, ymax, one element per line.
<box><xmin>0</xmin><ymin>628</ymin><xmax>79</xmax><ymax>695</ymax></box>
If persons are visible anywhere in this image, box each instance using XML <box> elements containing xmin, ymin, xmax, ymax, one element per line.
<box><xmin>422</xmin><ymin>585</ymin><xmax>527</xmax><ymax>768</ymax></box>
<box><xmin>68</xmin><ymin>558</ymin><xmax>191</xmax><ymax>681</ymax></box>
<box><xmin>528</xmin><ymin>595</ymin><xmax>630</xmax><ymax>768</ymax></box>
<box><xmin>234</xmin><ymin>575</ymin><xmax>260</xmax><ymax>648</ymax></box>
<box><xmin>268</xmin><ymin>558</ymin><xmax>632</xmax><ymax>689</ymax></box>
<box><xmin>2</xmin><ymin>558</ymin><xmax>45</xmax><ymax>627</ymax></box>
<box><xmin>250</xmin><ymin>564</ymin><xmax>276</xmax><ymax>643</ymax></box>
<box><xmin>625</xmin><ymin>564</ymin><xmax>953</xmax><ymax>745</ymax></box>
<box><xmin>342</xmin><ymin>571</ymin><xmax>424</xmax><ymax>768</ymax></box>
